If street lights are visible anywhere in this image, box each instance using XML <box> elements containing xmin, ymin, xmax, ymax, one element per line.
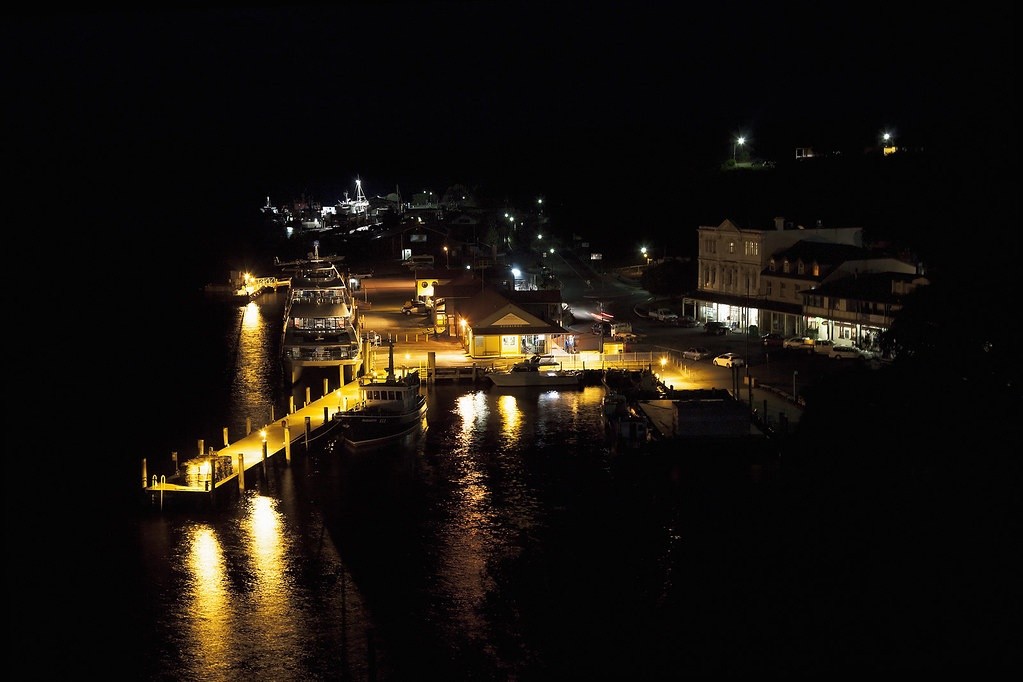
<box><xmin>443</xmin><ymin>246</ymin><xmax>450</xmax><ymax>271</ymax></box>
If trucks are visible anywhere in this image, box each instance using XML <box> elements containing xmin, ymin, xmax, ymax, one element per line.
<box><xmin>611</xmin><ymin>322</ymin><xmax>648</xmax><ymax>344</ymax></box>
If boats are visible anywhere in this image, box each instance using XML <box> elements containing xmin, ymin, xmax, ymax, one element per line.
<box><xmin>332</xmin><ymin>339</ymin><xmax>429</xmax><ymax>464</ymax></box>
<box><xmin>279</xmin><ymin>240</ymin><xmax>365</xmax><ymax>387</ymax></box>
<box><xmin>197</xmin><ymin>269</ymin><xmax>268</xmax><ymax>303</ymax></box>
<box><xmin>600</xmin><ymin>368</ymin><xmax>660</xmax><ymax>418</ymax></box>
<box><xmin>485</xmin><ymin>365</ymin><xmax>585</xmax><ymax>387</ymax></box>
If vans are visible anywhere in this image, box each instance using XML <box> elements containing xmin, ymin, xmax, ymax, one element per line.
<box><xmin>402</xmin><ymin>255</ymin><xmax>435</xmax><ymax>267</ymax></box>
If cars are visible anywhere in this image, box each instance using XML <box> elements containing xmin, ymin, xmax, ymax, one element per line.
<box><xmin>671</xmin><ymin>316</ymin><xmax>701</xmax><ymax>328</ymax></box>
<box><xmin>409</xmin><ymin>264</ymin><xmax>435</xmax><ymax>273</ymax></box>
<box><xmin>828</xmin><ymin>344</ymin><xmax>873</xmax><ymax>361</ymax></box>
<box><xmin>783</xmin><ymin>336</ymin><xmax>805</xmax><ymax>350</ymax></box>
<box><xmin>349</xmin><ymin>271</ymin><xmax>373</xmax><ymax>279</ymax></box>
<box><xmin>712</xmin><ymin>352</ymin><xmax>744</xmax><ymax>369</ymax></box>
<box><xmin>760</xmin><ymin>332</ymin><xmax>784</xmax><ymax>347</ymax></box>
<box><xmin>591</xmin><ymin>321</ymin><xmax>611</xmax><ymax>338</ymax></box>
<box><xmin>682</xmin><ymin>346</ymin><xmax>712</xmax><ymax>361</ymax></box>
<box><xmin>703</xmin><ymin>322</ymin><xmax>730</xmax><ymax>336</ymax></box>
<box><xmin>401</xmin><ymin>303</ymin><xmax>433</xmax><ymax>316</ymax></box>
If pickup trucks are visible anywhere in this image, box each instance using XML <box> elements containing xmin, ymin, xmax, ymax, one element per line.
<box><xmin>648</xmin><ymin>307</ymin><xmax>678</xmax><ymax>323</ymax></box>
<box><xmin>798</xmin><ymin>340</ymin><xmax>837</xmax><ymax>355</ymax></box>
